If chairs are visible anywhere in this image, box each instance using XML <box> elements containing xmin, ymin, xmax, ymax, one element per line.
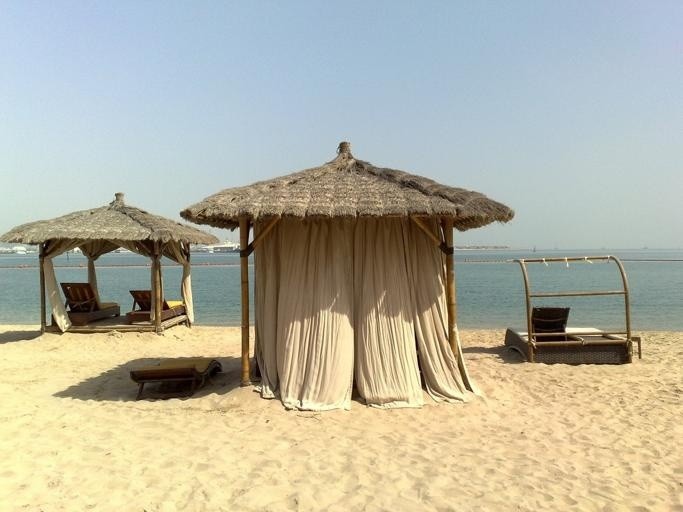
<box><xmin>52</xmin><ymin>283</ymin><xmax>120</xmax><ymax>326</ymax></box>
<box><xmin>531</xmin><ymin>306</ymin><xmax>570</xmax><ymax>342</ymax></box>
<box><xmin>130</xmin><ymin>358</ymin><xmax>223</xmax><ymax>400</ymax></box>
<box><xmin>126</xmin><ymin>290</ymin><xmax>184</xmax><ymax>325</ymax></box>
